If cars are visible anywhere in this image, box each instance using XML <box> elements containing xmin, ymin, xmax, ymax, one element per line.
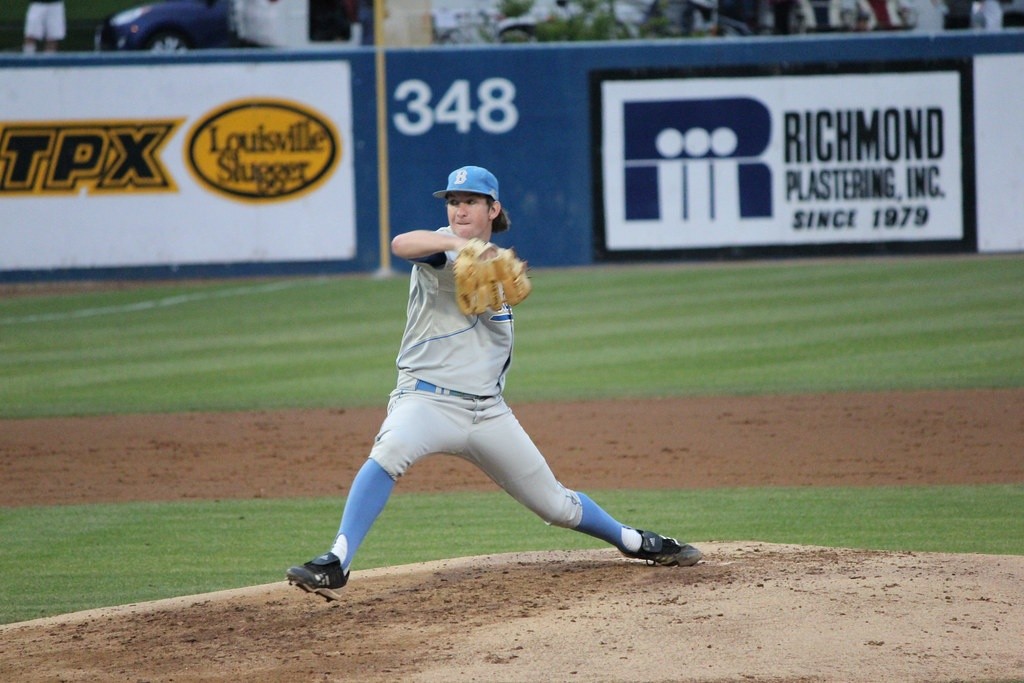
<box><xmin>98</xmin><ymin>0</ymin><xmax>375</xmax><ymax>53</ymax></box>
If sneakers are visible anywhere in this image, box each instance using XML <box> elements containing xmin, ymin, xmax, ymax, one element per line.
<box><xmin>622</xmin><ymin>529</ymin><xmax>702</xmax><ymax>566</ymax></box>
<box><xmin>286</xmin><ymin>552</ymin><xmax>350</xmax><ymax>602</ymax></box>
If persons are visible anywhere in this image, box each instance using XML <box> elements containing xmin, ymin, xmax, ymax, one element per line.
<box><xmin>286</xmin><ymin>165</ymin><xmax>702</xmax><ymax>604</ymax></box>
<box><xmin>647</xmin><ymin>0</ymin><xmax>1004</xmax><ymax>37</ymax></box>
<box><xmin>24</xmin><ymin>0</ymin><xmax>67</xmax><ymax>52</ymax></box>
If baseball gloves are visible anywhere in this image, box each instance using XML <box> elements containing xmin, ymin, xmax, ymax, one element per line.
<box><xmin>452</xmin><ymin>238</ymin><xmax>534</xmax><ymax>316</ymax></box>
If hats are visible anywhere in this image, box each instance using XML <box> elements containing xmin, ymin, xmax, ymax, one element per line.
<box><xmin>433</xmin><ymin>166</ymin><xmax>499</xmax><ymax>200</ymax></box>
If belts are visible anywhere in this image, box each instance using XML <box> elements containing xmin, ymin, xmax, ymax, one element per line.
<box><xmin>417</xmin><ymin>381</ymin><xmax>465</xmax><ymax>397</ymax></box>
